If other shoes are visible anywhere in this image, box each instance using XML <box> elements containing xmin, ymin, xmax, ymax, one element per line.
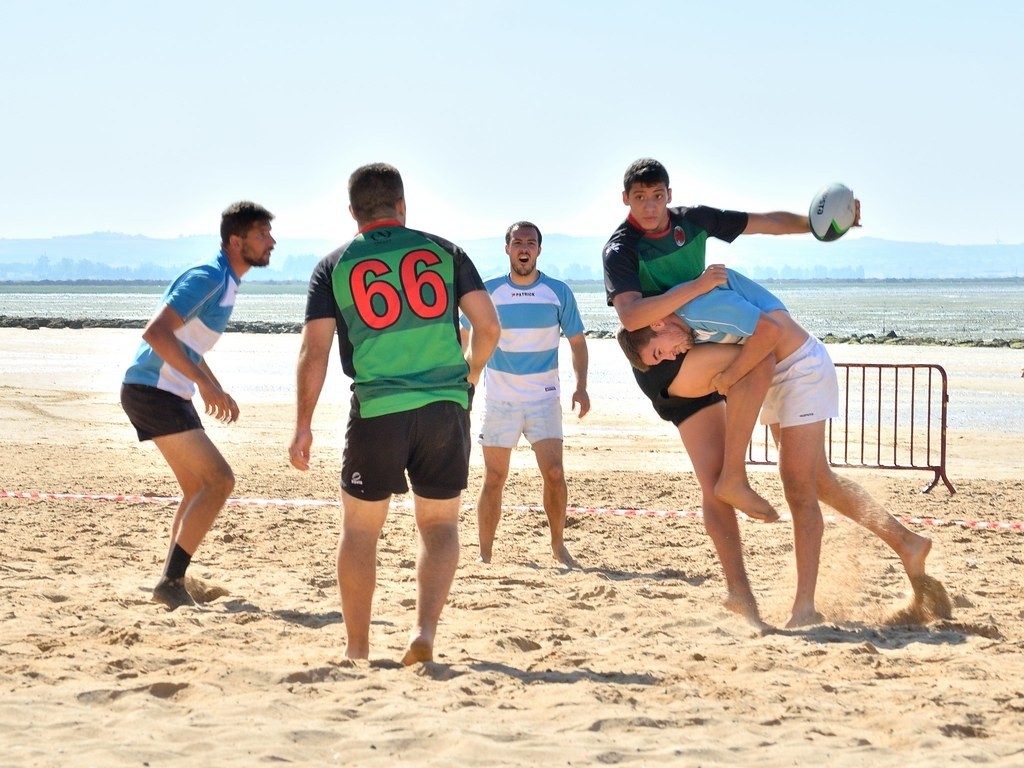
<box><xmin>152</xmin><ymin>578</ymin><xmax>197</xmax><ymax>610</ymax></box>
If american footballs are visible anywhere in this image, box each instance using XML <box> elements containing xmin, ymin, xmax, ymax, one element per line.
<box><xmin>807</xmin><ymin>182</ymin><xmax>856</xmax><ymax>243</ymax></box>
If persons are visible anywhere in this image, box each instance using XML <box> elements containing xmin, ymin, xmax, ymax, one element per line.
<box><xmin>601</xmin><ymin>158</ymin><xmax>862</xmax><ymax>632</ymax></box>
<box><xmin>617</xmin><ymin>267</ymin><xmax>932</xmax><ymax>625</ymax></box>
<box><xmin>458</xmin><ymin>220</ymin><xmax>591</xmax><ymax>570</ymax></box>
<box><xmin>287</xmin><ymin>163</ymin><xmax>502</xmax><ymax>669</ymax></box>
<box><xmin>122</xmin><ymin>202</ymin><xmax>278</xmax><ymax>609</ymax></box>
